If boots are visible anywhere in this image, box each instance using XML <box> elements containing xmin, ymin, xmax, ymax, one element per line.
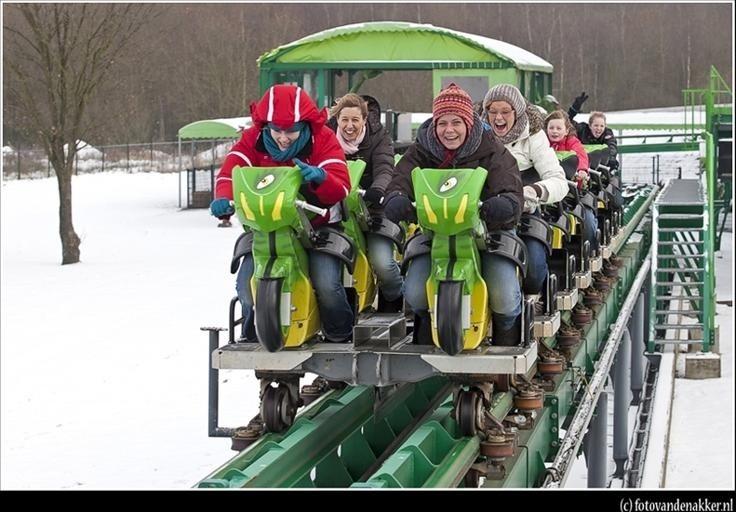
<box><xmin>491</xmin><ymin>314</ymin><xmax>520</xmax><ymax>346</ymax></box>
<box><xmin>412</xmin><ymin>309</ymin><xmax>432</xmax><ymax>344</ymax></box>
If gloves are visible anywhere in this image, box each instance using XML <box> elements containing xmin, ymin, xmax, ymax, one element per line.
<box><xmin>210</xmin><ymin>198</ymin><xmax>234</xmax><ymax>217</ymax></box>
<box><xmin>573</xmin><ymin>92</ymin><xmax>588</xmax><ymax>110</ymax></box>
<box><xmin>522</xmin><ymin>186</ymin><xmax>539</xmax><ymax>212</ymax></box>
<box><xmin>385</xmin><ymin>192</ymin><xmax>417</xmax><ymax>223</ymax></box>
<box><xmin>483</xmin><ymin>197</ymin><xmax>514</xmax><ymax>221</ymax></box>
<box><xmin>293</xmin><ymin>159</ymin><xmax>325</xmax><ymax>183</ymax></box>
<box><xmin>364</xmin><ymin>190</ymin><xmax>383</xmax><ymax>201</ymax></box>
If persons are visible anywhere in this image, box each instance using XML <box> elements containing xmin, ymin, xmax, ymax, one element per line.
<box><xmin>544</xmin><ymin>109</ymin><xmax>599</xmax><ymax>253</ymax></box>
<box><xmin>325</xmin><ymin>92</ymin><xmax>404</xmax><ymax>315</ymax></box>
<box><xmin>567</xmin><ymin>91</ymin><xmax>625</xmax><ymax>210</ymax></box>
<box><xmin>382</xmin><ymin>84</ymin><xmax>526</xmax><ymax>347</ymax></box>
<box><xmin>472</xmin><ymin>84</ymin><xmax>570</xmax><ymax>294</ymax></box>
<box><xmin>209</xmin><ymin>84</ymin><xmax>354</xmax><ymax>344</ymax></box>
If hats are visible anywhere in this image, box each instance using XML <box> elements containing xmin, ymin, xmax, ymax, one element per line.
<box><xmin>484</xmin><ymin>84</ymin><xmax>526</xmax><ymax>117</ymax></box>
<box><xmin>433</xmin><ymin>83</ymin><xmax>474</xmax><ymax>135</ymax></box>
<box><xmin>269</xmin><ymin>121</ymin><xmax>305</xmax><ymax>131</ymax></box>
